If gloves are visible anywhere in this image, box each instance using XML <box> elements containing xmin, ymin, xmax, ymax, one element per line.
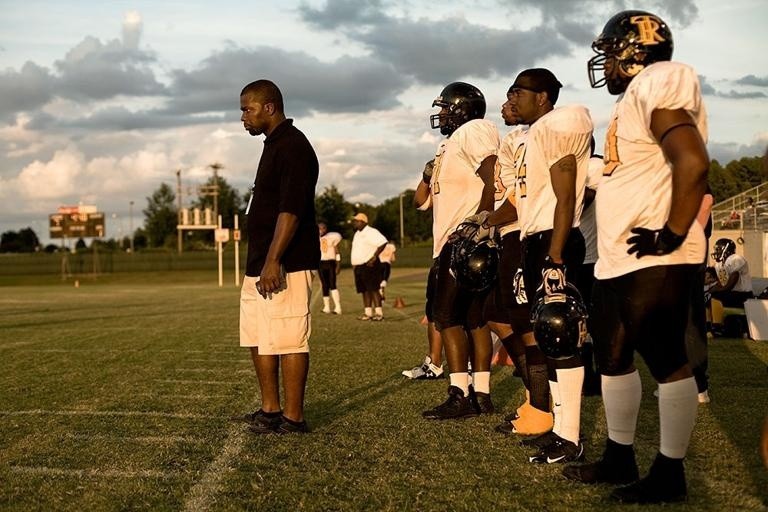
<box><xmin>513</xmin><ymin>269</ymin><xmax>529</xmax><ymax>306</ymax></box>
<box><xmin>626</xmin><ymin>220</ymin><xmax>687</xmax><ymax>259</ymax></box>
<box><xmin>463</xmin><ymin>210</ymin><xmax>497</xmax><ymax>244</ymax></box>
<box><xmin>536</xmin><ymin>255</ymin><xmax>567</xmax><ymax>304</ymax></box>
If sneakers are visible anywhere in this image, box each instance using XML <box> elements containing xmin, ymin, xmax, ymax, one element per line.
<box><xmin>232</xmin><ymin>407</ymin><xmax>283</xmax><ymax>423</ymax></box>
<box><xmin>358</xmin><ymin>315</ymin><xmax>369</xmax><ymax>321</ymax></box>
<box><xmin>253</xmin><ymin>417</ymin><xmax>308</xmax><ymax>435</ymax></box>
<box><xmin>372</xmin><ymin>315</ymin><xmax>382</xmax><ymax>320</ymax></box>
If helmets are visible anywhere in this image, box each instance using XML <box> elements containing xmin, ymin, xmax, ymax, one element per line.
<box><xmin>588</xmin><ymin>10</ymin><xmax>674</xmax><ymax>95</ymax></box>
<box><xmin>531</xmin><ymin>283</ymin><xmax>589</xmax><ymax>361</ymax></box>
<box><xmin>711</xmin><ymin>237</ymin><xmax>736</xmax><ymax>261</ymax></box>
<box><xmin>430</xmin><ymin>82</ymin><xmax>485</xmax><ymax>135</ymax></box>
<box><xmin>439</xmin><ymin>222</ymin><xmax>508</xmax><ymax>291</ymax></box>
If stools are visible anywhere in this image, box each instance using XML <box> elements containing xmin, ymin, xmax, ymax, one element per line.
<box><xmin>725</xmin><ymin>306</ymin><xmax>744</xmax><ymax>321</ymax></box>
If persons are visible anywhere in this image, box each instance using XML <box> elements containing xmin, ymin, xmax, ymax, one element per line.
<box><xmin>351</xmin><ymin>213</ymin><xmax>389</xmax><ymax>321</ymax></box>
<box><xmin>400</xmin><ymin>84</ymin><xmax>606</xmax><ymax>441</ymax></box>
<box><xmin>227</xmin><ymin>79</ymin><xmax>324</xmax><ymax>438</ymax></box>
<box><xmin>318</xmin><ymin>222</ymin><xmax>343</xmax><ymax>315</ymax></box>
<box><xmin>703</xmin><ymin>239</ymin><xmax>755</xmax><ymax>339</ymax></box>
<box><xmin>564</xmin><ymin>11</ymin><xmax>706</xmax><ymax>504</ymax></box>
<box><xmin>654</xmin><ymin>183</ymin><xmax>710</xmax><ymax>405</ymax></box>
<box><xmin>378</xmin><ymin>243</ymin><xmax>396</xmax><ymax>287</ymax></box>
<box><xmin>510</xmin><ymin>68</ymin><xmax>587</xmax><ymax>464</ymax></box>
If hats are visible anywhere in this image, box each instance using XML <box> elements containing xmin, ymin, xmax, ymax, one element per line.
<box><xmin>354</xmin><ymin>213</ymin><xmax>369</xmax><ymax>224</ymax></box>
<box><xmin>514</xmin><ymin>68</ymin><xmax>563</xmax><ymax>104</ymax></box>
